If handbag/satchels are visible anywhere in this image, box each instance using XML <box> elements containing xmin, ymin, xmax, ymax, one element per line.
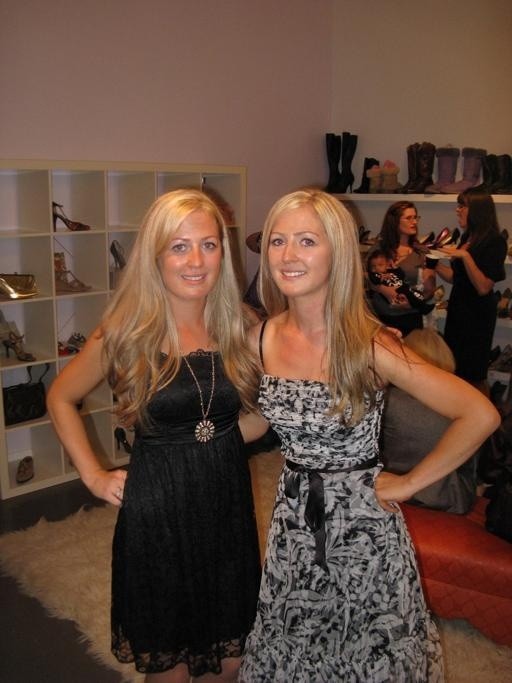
<box><xmin>0</xmin><ymin>272</ymin><xmax>35</xmax><ymax>300</ymax></box>
<box><xmin>2</xmin><ymin>381</ymin><xmax>47</xmax><ymax>426</ymax></box>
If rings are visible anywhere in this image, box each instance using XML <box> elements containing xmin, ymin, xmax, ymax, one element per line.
<box><xmin>116</xmin><ymin>488</ymin><xmax>121</xmax><ymax>498</ymax></box>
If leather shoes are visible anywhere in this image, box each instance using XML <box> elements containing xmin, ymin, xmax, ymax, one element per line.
<box><xmin>489</xmin><ymin>343</ymin><xmax>511</xmax><ymax>372</ymax></box>
<box><xmin>16</xmin><ymin>455</ymin><xmax>34</xmax><ymax>484</ymax></box>
<box><xmin>495</xmin><ymin>287</ymin><xmax>511</xmax><ymax>318</ymax></box>
<box><xmin>0</xmin><ymin>277</ymin><xmax>39</xmax><ymax>298</ymax></box>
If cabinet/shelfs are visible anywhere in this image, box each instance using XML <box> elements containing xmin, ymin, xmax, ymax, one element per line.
<box><xmin>327</xmin><ymin>192</ymin><xmax>511</xmax><ymax>384</ymax></box>
<box><xmin>0</xmin><ymin>158</ymin><xmax>248</xmax><ymax>502</ymax></box>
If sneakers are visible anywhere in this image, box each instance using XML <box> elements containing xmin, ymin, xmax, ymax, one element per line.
<box><xmin>67</xmin><ymin>333</ymin><xmax>87</xmax><ymax>346</ymax></box>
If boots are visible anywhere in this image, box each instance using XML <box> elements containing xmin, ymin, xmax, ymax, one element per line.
<box><xmin>323</xmin><ymin>131</ymin><xmax>358</xmax><ymax>194</ymax></box>
<box><xmin>353</xmin><ymin>157</ymin><xmax>404</xmax><ymax>194</ymax></box>
<box><xmin>393</xmin><ymin>141</ymin><xmax>512</xmax><ymax>195</ymax></box>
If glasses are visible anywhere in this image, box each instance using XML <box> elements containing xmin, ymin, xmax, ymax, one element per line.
<box><xmin>400</xmin><ymin>215</ymin><xmax>422</xmax><ymax>222</ymax></box>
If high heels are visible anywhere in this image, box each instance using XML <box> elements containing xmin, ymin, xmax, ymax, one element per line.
<box><xmin>114</xmin><ymin>426</ymin><xmax>131</xmax><ymax>454</ymax></box>
<box><xmin>419</xmin><ymin>227</ymin><xmax>460</xmax><ymax>250</ymax></box>
<box><xmin>110</xmin><ymin>239</ymin><xmax>126</xmax><ymax>271</ymax></box>
<box><xmin>3</xmin><ymin>331</ymin><xmax>37</xmax><ymax>362</ymax></box>
<box><xmin>52</xmin><ymin>201</ymin><xmax>91</xmax><ymax>232</ymax></box>
<box><xmin>56</xmin><ymin>270</ymin><xmax>92</xmax><ymax>292</ymax></box>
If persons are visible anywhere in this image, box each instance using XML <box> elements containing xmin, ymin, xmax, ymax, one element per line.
<box><xmin>436</xmin><ymin>186</ymin><xmax>508</xmax><ymax>398</ymax></box>
<box><xmin>237</xmin><ymin>186</ymin><xmax>502</xmax><ymax>683</ymax></box>
<box><xmin>365</xmin><ymin>199</ymin><xmax>438</xmax><ymax>338</ymax></box>
<box><xmin>46</xmin><ymin>187</ymin><xmax>263</xmax><ymax>683</ymax></box>
<box><xmin>377</xmin><ymin>328</ymin><xmax>478</xmax><ymax>515</ymax></box>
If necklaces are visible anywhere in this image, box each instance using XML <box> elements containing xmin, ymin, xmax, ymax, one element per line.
<box><xmin>177</xmin><ymin>344</ymin><xmax>216</xmax><ymax>443</ymax></box>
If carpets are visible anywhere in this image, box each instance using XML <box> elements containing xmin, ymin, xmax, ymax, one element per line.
<box><xmin>1</xmin><ymin>463</ymin><xmax>132</xmax><ymax>682</ymax></box>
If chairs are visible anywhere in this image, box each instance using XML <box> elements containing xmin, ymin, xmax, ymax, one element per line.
<box><xmin>398</xmin><ymin>468</ymin><xmax>511</xmax><ymax>647</ymax></box>
